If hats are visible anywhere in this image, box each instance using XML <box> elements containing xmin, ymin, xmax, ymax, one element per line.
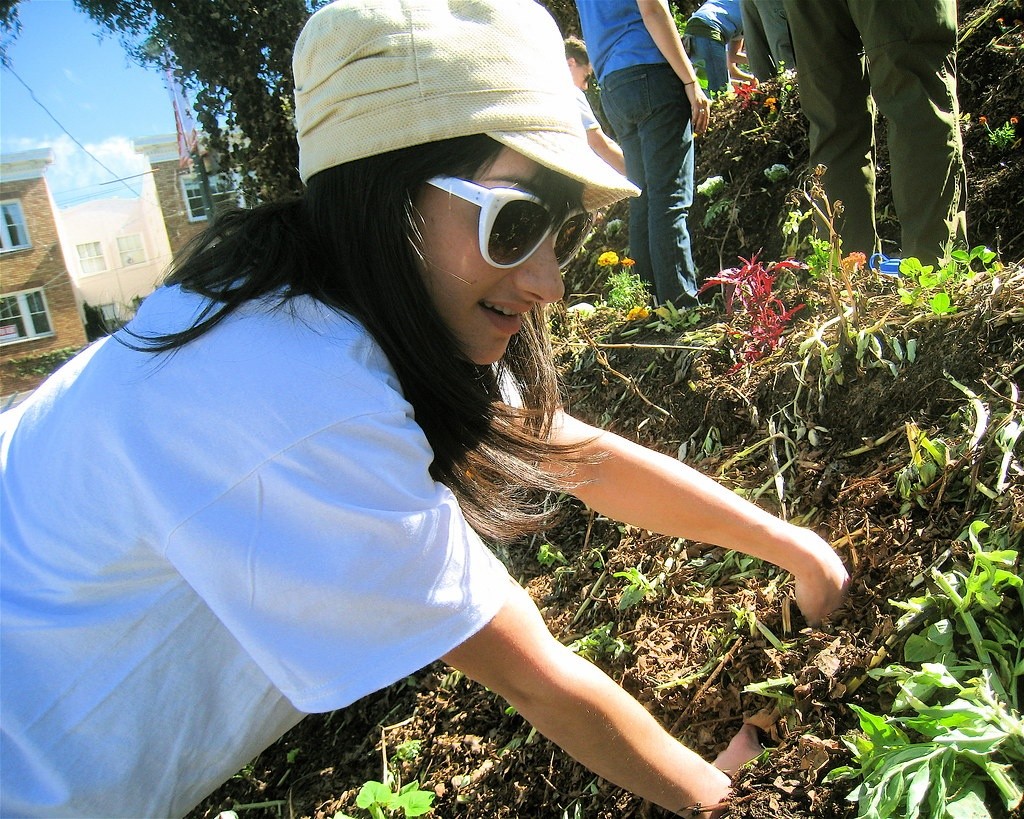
<box><xmin>292</xmin><ymin>0</ymin><xmax>643</xmax><ymax>212</ymax></box>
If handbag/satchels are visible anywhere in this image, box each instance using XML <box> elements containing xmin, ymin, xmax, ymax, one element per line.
<box><xmin>681</xmin><ymin>35</ymin><xmax>691</xmax><ymax>58</ymax></box>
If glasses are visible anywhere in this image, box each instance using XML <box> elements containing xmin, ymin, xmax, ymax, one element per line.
<box><xmin>576</xmin><ymin>59</ymin><xmax>591</xmax><ymax>82</ymax></box>
<box><xmin>426</xmin><ymin>174</ymin><xmax>597</xmax><ymax>269</ymax></box>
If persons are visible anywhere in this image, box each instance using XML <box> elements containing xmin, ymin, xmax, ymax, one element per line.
<box><xmin>559</xmin><ymin>0</ymin><xmax>971</xmax><ymax>305</ymax></box>
<box><xmin>0</xmin><ymin>0</ymin><xmax>854</xmax><ymax>817</ymax></box>
